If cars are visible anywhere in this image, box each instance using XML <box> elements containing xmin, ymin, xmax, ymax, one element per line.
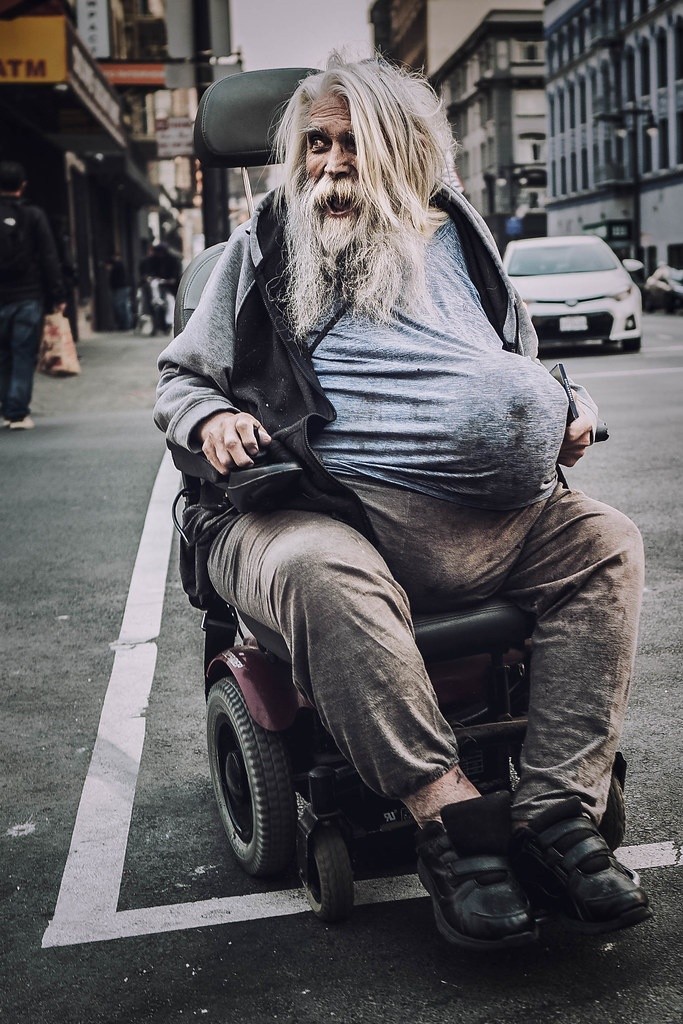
<box><xmin>502</xmin><ymin>235</ymin><xmax>641</xmax><ymax>352</ymax></box>
<box><xmin>644</xmin><ymin>266</ymin><xmax>683</xmax><ymax>309</ymax></box>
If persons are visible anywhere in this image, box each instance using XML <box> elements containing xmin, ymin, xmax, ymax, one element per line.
<box><xmin>0</xmin><ymin>164</ymin><xmax>66</xmax><ymax>430</ymax></box>
<box><xmin>155</xmin><ymin>60</ymin><xmax>652</xmax><ymax>945</ymax></box>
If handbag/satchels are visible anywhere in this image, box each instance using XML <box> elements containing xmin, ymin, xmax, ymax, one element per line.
<box><xmin>38</xmin><ymin>309</ymin><xmax>82</xmax><ymax>377</ymax></box>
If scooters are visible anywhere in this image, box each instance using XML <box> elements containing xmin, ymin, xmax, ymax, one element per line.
<box><xmin>177</xmin><ymin>66</ymin><xmax>642</xmax><ymax>927</ymax></box>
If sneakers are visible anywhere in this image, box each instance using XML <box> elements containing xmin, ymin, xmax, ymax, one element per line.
<box><xmin>514</xmin><ymin>795</ymin><xmax>654</xmax><ymax>935</ymax></box>
<box><xmin>415</xmin><ymin>790</ymin><xmax>540</xmax><ymax>952</ymax></box>
<box><xmin>0</xmin><ymin>417</ymin><xmax>34</xmax><ymax>430</ymax></box>
